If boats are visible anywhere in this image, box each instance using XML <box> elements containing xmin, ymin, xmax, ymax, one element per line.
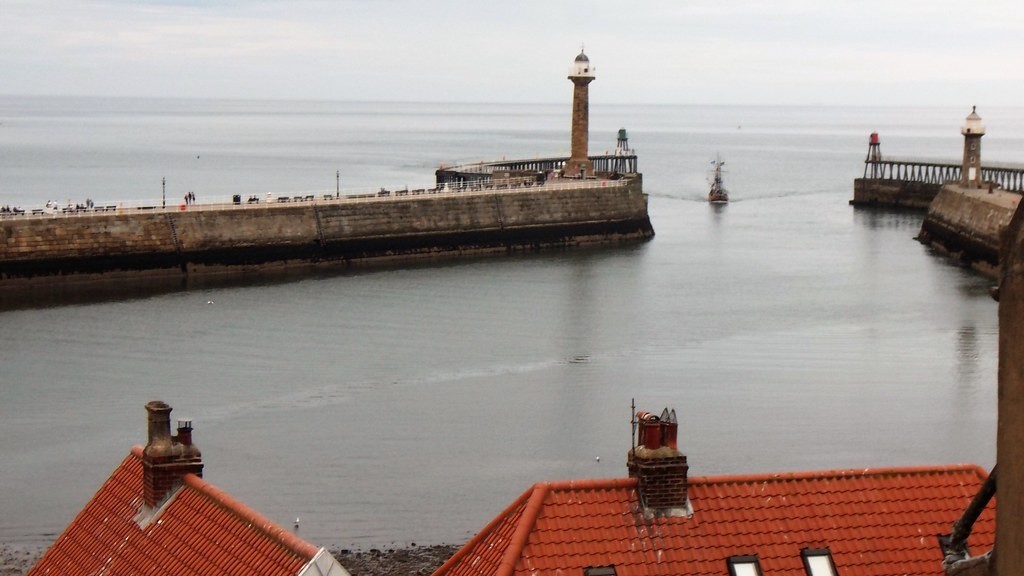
<box><xmin>705</xmin><ymin>152</ymin><xmax>729</xmax><ymax>205</ymax></box>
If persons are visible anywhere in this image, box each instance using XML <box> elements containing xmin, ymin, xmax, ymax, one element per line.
<box><xmin>543</xmin><ymin>168</ymin><xmax>566</xmax><ymax>179</ymax></box>
<box><xmin>2</xmin><ymin>205</ymin><xmax>21</xmax><ymax>215</ymax></box>
<box><xmin>184</xmin><ymin>192</ymin><xmax>195</xmax><ymax>205</ymax></box>
<box><xmin>47</xmin><ymin>198</ymin><xmax>94</xmax><ymax>213</ymax></box>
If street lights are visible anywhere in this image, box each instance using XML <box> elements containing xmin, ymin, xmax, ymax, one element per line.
<box><xmin>337</xmin><ymin>170</ymin><xmax>339</xmax><ymax>200</ymax></box>
<box><xmin>162</xmin><ymin>175</ymin><xmax>166</xmax><ymax>208</ymax></box>
